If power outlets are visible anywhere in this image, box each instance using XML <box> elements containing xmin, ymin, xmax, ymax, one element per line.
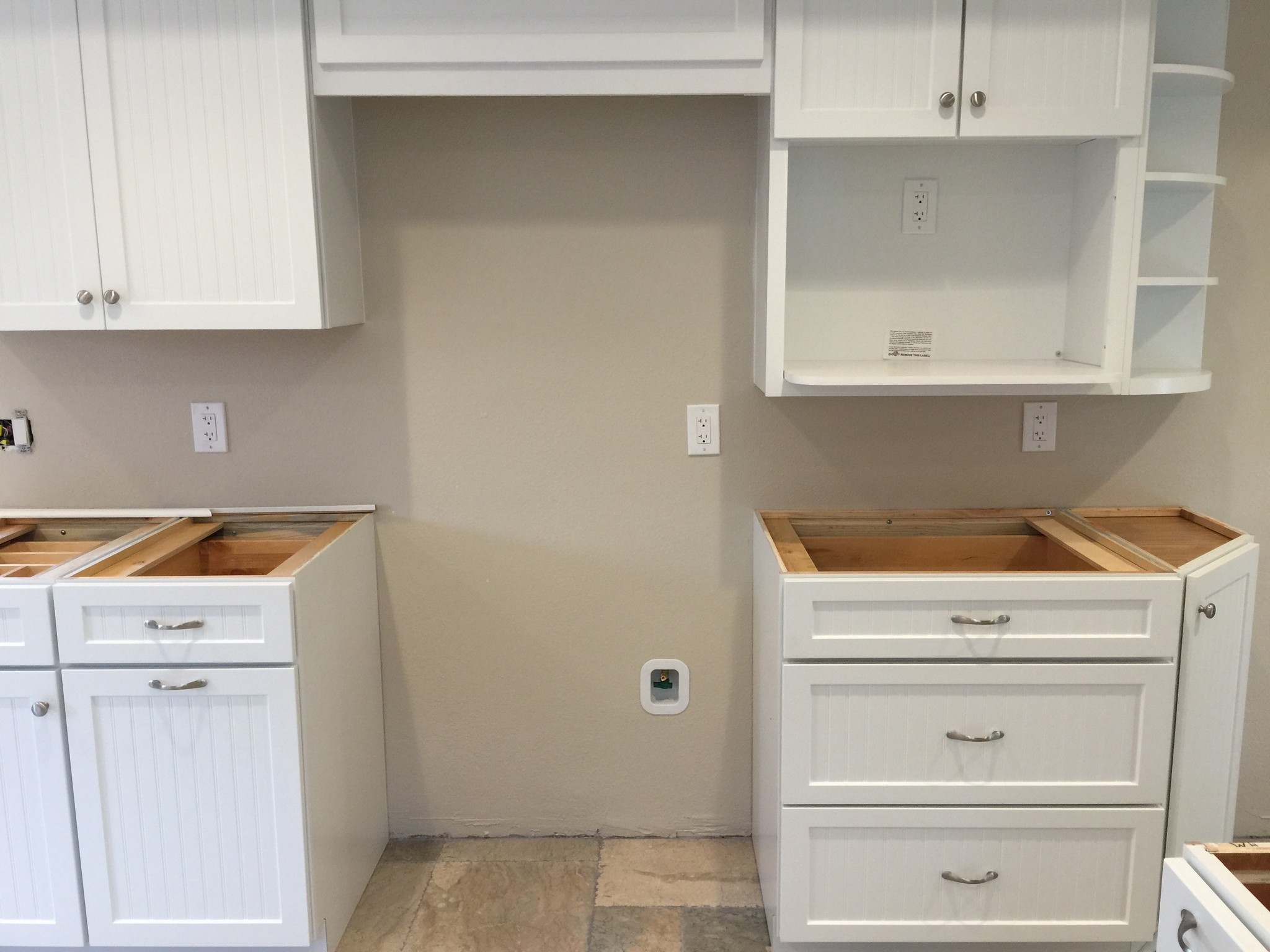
<box><xmin>1021</xmin><ymin>400</ymin><xmax>1058</xmax><ymax>452</ymax></box>
<box><xmin>901</xmin><ymin>179</ymin><xmax>937</xmax><ymax>234</ymax></box>
<box><xmin>687</xmin><ymin>405</ymin><xmax>721</xmax><ymax>458</ymax></box>
<box><xmin>191</xmin><ymin>403</ymin><xmax>230</xmax><ymax>454</ymax></box>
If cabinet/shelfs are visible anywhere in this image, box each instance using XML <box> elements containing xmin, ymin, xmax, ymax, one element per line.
<box><xmin>779</xmin><ymin>575</ymin><xmax>1185</xmax><ymax>952</ymax></box>
<box><xmin>1125</xmin><ymin>61</ymin><xmax>1236</xmax><ymax>395</ymax></box>
<box><xmin>753</xmin><ymin>0</ymin><xmax>1151</xmax><ymax>399</ymax></box>
<box><xmin>51</xmin><ymin>577</ymin><xmax>314</xmax><ymax>952</ymax></box>
<box><xmin>0</xmin><ymin>0</ymin><xmax>365</xmax><ymax>331</ymax></box>
<box><xmin>0</xmin><ymin>584</ymin><xmax>89</xmax><ymax>952</ymax></box>
<box><xmin>1165</xmin><ymin>542</ymin><xmax>1261</xmax><ymax>854</ymax></box>
<box><xmin>305</xmin><ymin>0</ymin><xmax>776</xmax><ymax>98</ymax></box>
<box><xmin>1154</xmin><ymin>854</ymin><xmax>1269</xmax><ymax>952</ymax></box>
